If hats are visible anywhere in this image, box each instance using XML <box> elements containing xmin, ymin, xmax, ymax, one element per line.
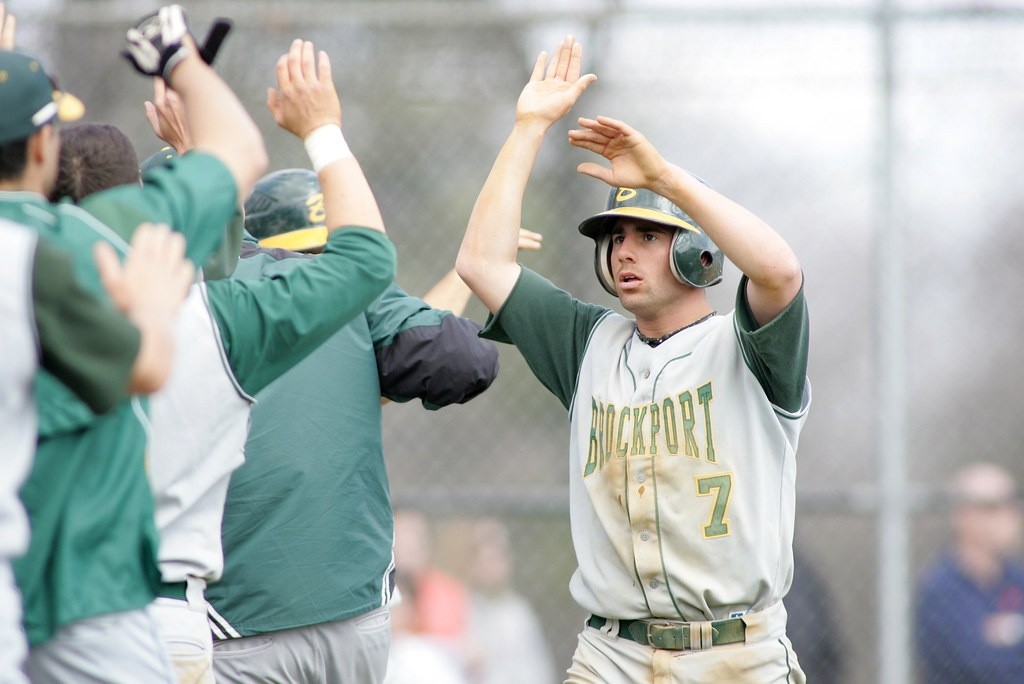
<box><xmin>0</xmin><ymin>49</ymin><xmax>85</xmax><ymax>143</ymax></box>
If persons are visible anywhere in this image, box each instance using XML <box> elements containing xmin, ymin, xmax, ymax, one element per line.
<box><xmin>453</xmin><ymin>33</ymin><xmax>815</xmax><ymax>684</ymax></box>
<box><xmin>909</xmin><ymin>460</ymin><xmax>1024</xmax><ymax>684</ymax></box>
<box><xmin>0</xmin><ymin>3</ymin><xmax>544</xmax><ymax>684</ymax></box>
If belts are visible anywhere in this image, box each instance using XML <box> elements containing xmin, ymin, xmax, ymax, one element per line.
<box><xmin>588</xmin><ymin>612</ymin><xmax>745</xmax><ymax>650</ymax></box>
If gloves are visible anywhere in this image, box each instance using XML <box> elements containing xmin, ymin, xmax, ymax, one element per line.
<box><xmin>124</xmin><ymin>6</ymin><xmax>200</xmax><ymax>89</ymax></box>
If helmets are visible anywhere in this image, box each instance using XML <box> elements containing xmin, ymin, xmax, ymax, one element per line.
<box><xmin>577</xmin><ymin>185</ymin><xmax>724</xmax><ymax>296</ymax></box>
<box><xmin>241</xmin><ymin>168</ymin><xmax>330</xmax><ymax>253</ymax></box>
